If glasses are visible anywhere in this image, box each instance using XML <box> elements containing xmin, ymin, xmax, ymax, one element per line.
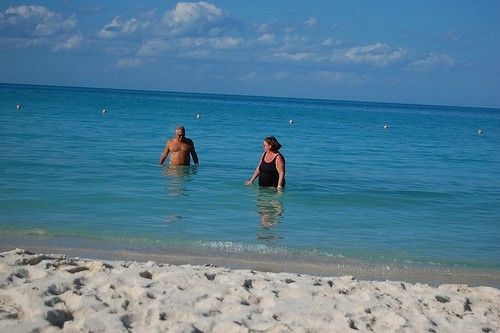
<box><xmin>263</xmin><ymin>144</ymin><xmax>269</xmax><ymax>147</ymax></box>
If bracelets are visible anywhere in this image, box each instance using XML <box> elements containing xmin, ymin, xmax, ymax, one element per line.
<box><xmin>195</xmin><ymin>163</ymin><xmax>199</xmax><ymax>166</ymax></box>
<box><xmin>278</xmin><ymin>185</ymin><xmax>282</xmax><ymax>188</ymax></box>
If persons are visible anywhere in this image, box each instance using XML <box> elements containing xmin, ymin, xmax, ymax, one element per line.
<box><xmin>159</xmin><ymin>126</ymin><xmax>199</xmax><ymax>167</ymax></box>
<box><xmin>244</xmin><ymin>136</ymin><xmax>286</xmax><ymax>189</ymax></box>
